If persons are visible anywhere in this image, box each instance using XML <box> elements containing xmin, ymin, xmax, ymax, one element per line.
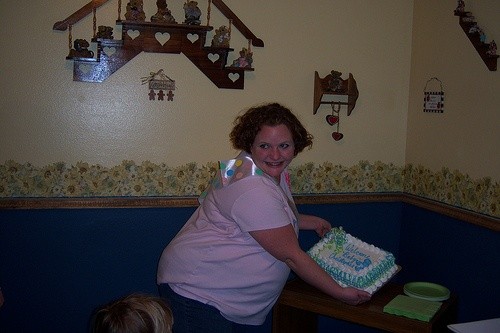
<box><xmin>157</xmin><ymin>103</ymin><xmax>371</xmax><ymax>333</ymax></box>
<box><xmin>90</xmin><ymin>294</ymin><xmax>173</xmax><ymax>333</ymax></box>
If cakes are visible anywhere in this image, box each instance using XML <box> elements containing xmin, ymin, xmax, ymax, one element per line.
<box><xmin>306</xmin><ymin>227</ymin><xmax>398</xmax><ymax>295</ymax></box>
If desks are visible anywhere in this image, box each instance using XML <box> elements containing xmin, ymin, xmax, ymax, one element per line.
<box><xmin>272</xmin><ymin>278</ymin><xmax>457</xmax><ymax>333</ymax></box>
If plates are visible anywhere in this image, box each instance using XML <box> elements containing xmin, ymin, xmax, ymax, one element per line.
<box><xmin>403</xmin><ymin>282</ymin><xmax>450</xmax><ymax>302</ymax></box>
